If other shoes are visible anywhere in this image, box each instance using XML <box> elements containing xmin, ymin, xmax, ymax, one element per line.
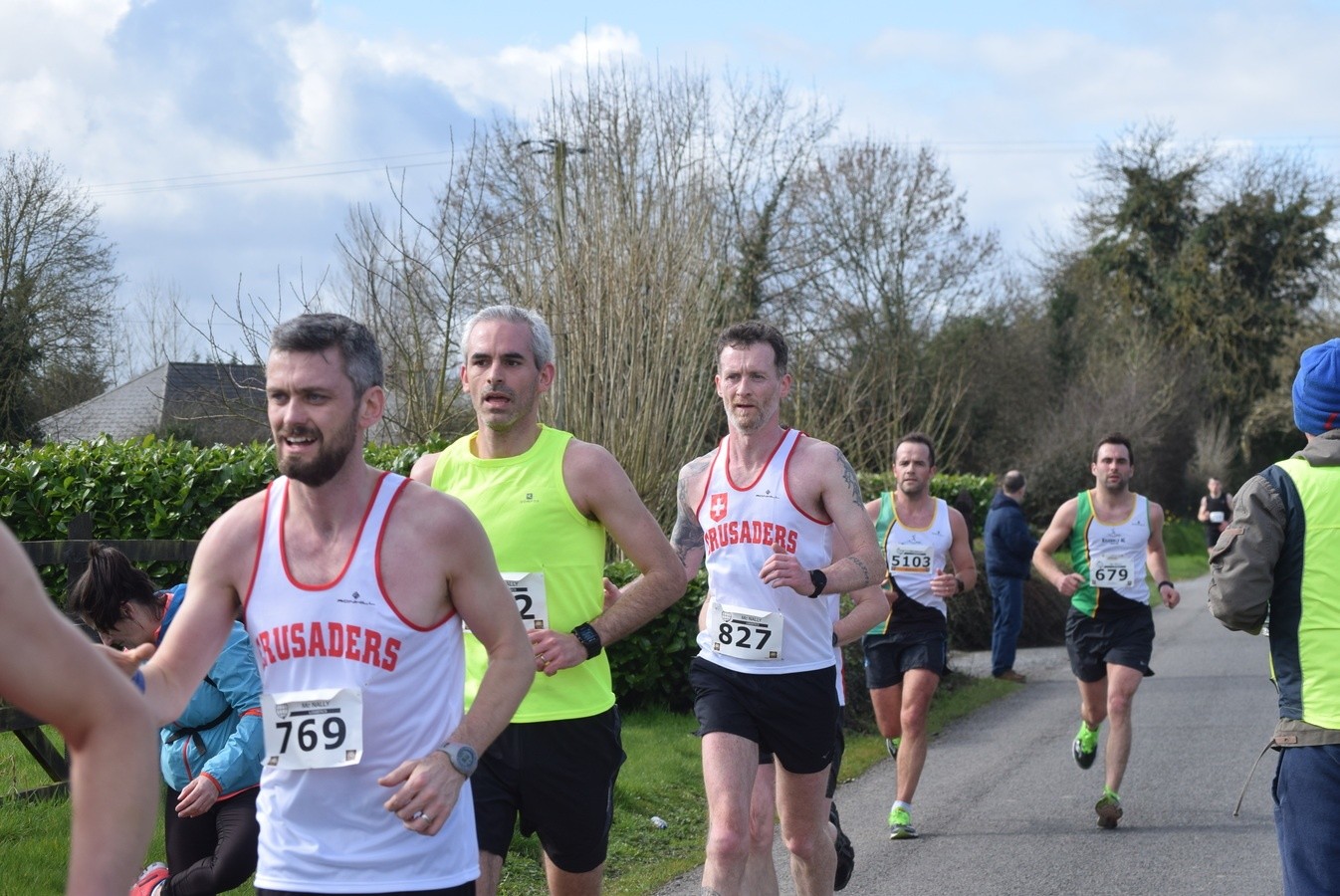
<box><xmin>999</xmin><ymin>669</ymin><xmax>1024</xmax><ymax>683</ymax></box>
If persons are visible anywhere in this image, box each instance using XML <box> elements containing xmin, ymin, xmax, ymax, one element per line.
<box><xmin>89</xmin><ymin>314</ymin><xmax>536</xmax><ymax>896</ymax></box>
<box><xmin>984</xmin><ymin>470</ymin><xmax>1040</xmax><ymax>682</ymax></box>
<box><xmin>603</xmin><ymin>322</ymin><xmax>891</xmax><ymax>895</ymax></box>
<box><xmin>860</xmin><ymin>432</ymin><xmax>976</xmax><ymax>839</ymax></box>
<box><xmin>1198</xmin><ymin>337</ymin><xmax>1340</xmax><ymax>896</ymax></box>
<box><xmin>1</xmin><ymin>522</ymin><xmax>265</xmax><ymax>896</ymax></box>
<box><xmin>1032</xmin><ymin>431</ymin><xmax>1180</xmax><ymax>830</ymax></box>
<box><xmin>407</xmin><ymin>304</ymin><xmax>687</xmax><ymax>895</ymax></box>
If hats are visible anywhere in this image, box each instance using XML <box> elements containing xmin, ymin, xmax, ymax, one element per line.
<box><xmin>1291</xmin><ymin>337</ymin><xmax>1340</xmax><ymax>435</ymax></box>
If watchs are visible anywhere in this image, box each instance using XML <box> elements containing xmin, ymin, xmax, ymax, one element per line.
<box><xmin>956</xmin><ymin>578</ymin><xmax>964</xmax><ymax>594</ymax></box>
<box><xmin>808</xmin><ymin>569</ymin><xmax>827</xmax><ymax>598</ymax></box>
<box><xmin>571</xmin><ymin>623</ymin><xmax>601</xmax><ymax>660</ymax></box>
<box><xmin>436</xmin><ymin>741</ymin><xmax>478</xmax><ymax>781</ymax></box>
<box><xmin>833</xmin><ymin>632</ymin><xmax>838</xmax><ymax>647</ymax></box>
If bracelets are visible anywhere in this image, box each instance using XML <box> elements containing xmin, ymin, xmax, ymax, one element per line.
<box><xmin>132</xmin><ymin>669</ymin><xmax>145</xmax><ymax>694</ymax></box>
<box><xmin>1158</xmin><ymin>581</ymin><xmax>1174</xmax><ymax>592</ymax></box>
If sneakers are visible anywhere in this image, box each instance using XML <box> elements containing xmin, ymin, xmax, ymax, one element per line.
<box><xmin>887</xmin><ymin>736</ymin><xmax>903</xmax><ymax>759</ymax></box>
<box><xmin>125</xmin><ymin>862</ymin><xmax>169</xmax><ymax>896</ymax></box>
<box><xmin>888</xmin><ymin>808</ymin><xmax>916</xmax><ymax>839</ymax></box>
<box><xmin>1095</xmin><ymin>786</ymin><xmax>1122</xmax><ymax>829</ymax></box>
<box><xmin>1072</xmin><ymin>719</ymin><xmax>1100</xmax><ymax>770</ymax></box>
<box><xmin>826</xmin><ymin>802</ymin><xmax>854</xmax><ymax>891</ymax></box>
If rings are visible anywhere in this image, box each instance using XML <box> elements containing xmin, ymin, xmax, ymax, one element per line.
<box><xmin>540</xmin><ymin>656</ymin><xmax>547</xmax><ymax>663</ymax></box>
<box><xmin>946</xmin><ymin>587</ymin><xmax>948</xmax><ymax>591</ymax></box>
<box><xmin>420</xmin><ymin>814</ymin><xmax>432</xmax><ymax>826</ymax></box>
